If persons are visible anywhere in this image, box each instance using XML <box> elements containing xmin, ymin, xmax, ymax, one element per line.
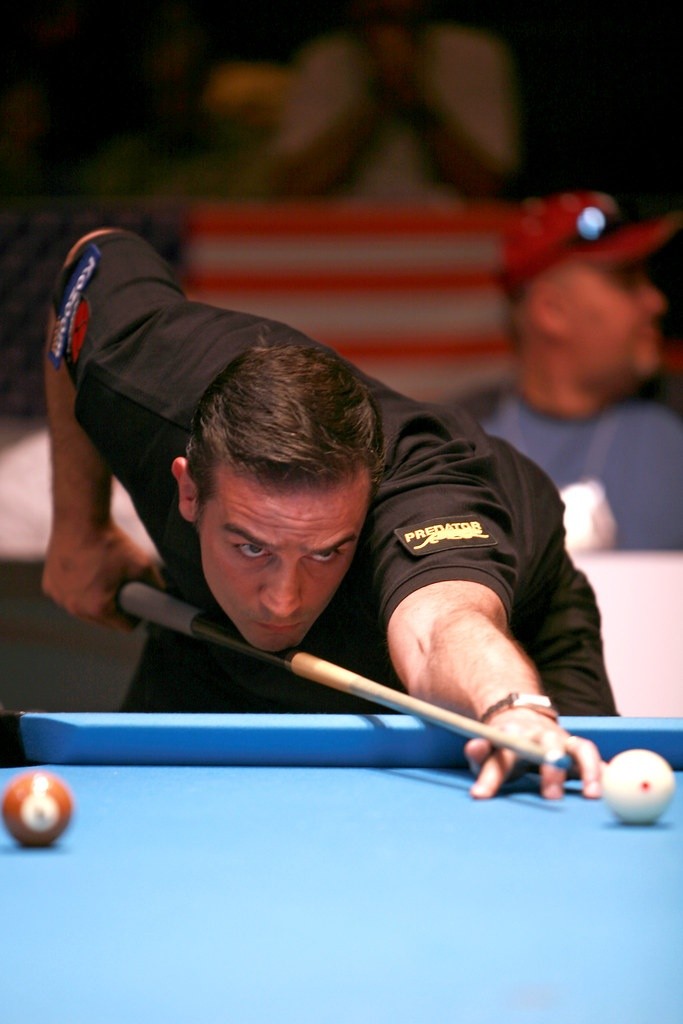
<box><xmin>36</xmin><ymin>227</ymin><xmax>619</xmax><ymax>801</ymax></box>
<box><xmin>0</xmin><ymin>0</ymin><xmax>682</xmax><ymax>563</ymax></box>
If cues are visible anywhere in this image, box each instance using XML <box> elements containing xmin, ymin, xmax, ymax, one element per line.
<box><xmin>113</xmin><ymin>576</ymin><xmax>573</xmax><ymax>769</ymax></box>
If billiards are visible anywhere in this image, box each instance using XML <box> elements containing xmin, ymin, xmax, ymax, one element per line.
<box><xmin>599</xmin><ymin>748</ymin><xmax>676</xmax><ymax>827</ymax></box>
<box><xmin>3</xmin><ymin>767</ymin><xmax>74</xmax><ymax>848</ymax></box>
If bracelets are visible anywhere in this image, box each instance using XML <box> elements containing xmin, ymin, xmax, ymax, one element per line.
<box><xmin>481</xmin><ymin>693</ymin><xmax>558</xmax><ymax>725</ymax></box>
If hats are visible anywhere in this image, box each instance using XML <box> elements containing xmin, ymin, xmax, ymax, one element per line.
<box><xmin>498</xmin><ymin>193</ymin><xmax>683</xmax><ymax>296</ymax></box>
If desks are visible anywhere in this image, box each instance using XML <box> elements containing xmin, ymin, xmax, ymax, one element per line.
<box><xmin>0</xmin><ymin>710</ymin><xmax>682</xmax><ymax>1024</ymax></box>
